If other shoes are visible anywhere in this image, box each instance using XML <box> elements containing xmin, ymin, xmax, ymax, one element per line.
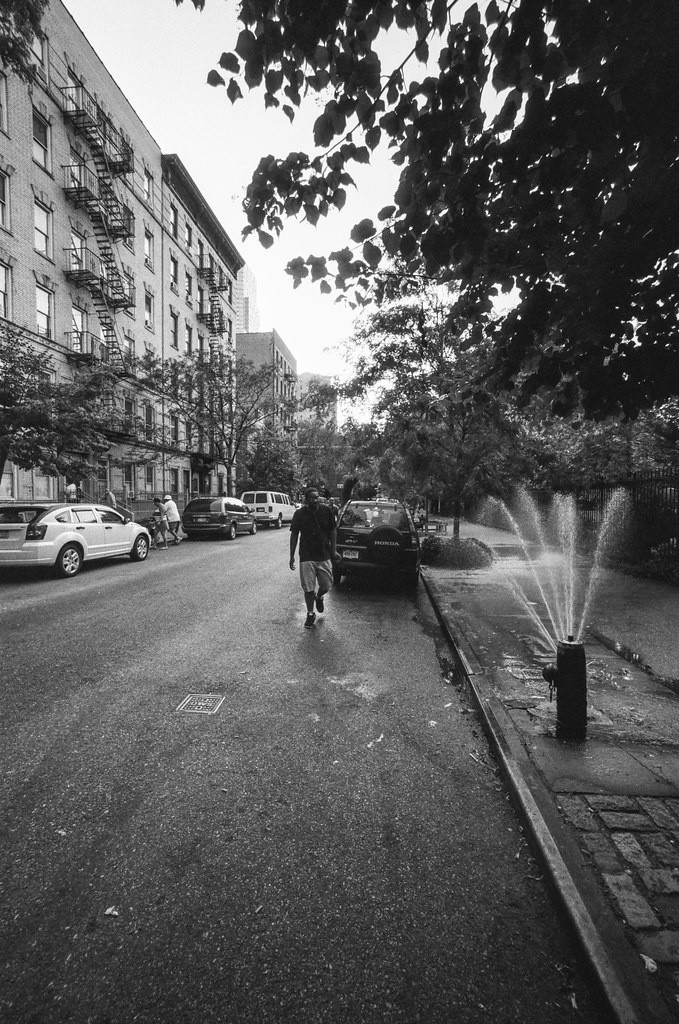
<box><xmin>305</xmin><ymin>611</ymin><xmax>316</xmax><ymax>628</ymax></box>
<box><xmin>314</xmin><ymin>593</ymin><xmax>324</xmax><ymax>613</ymax></box>
<box><xmin>160</xmin><ymin>545</ymin><xmax>168</xmax><ymax>549</ymax></box>
<box><xmin>150</xmin><ymin>545</ymin><xmax>157</xmax><ymax>549</ymax></box>
<box><xmin>176</xmin><ymin>537</ymin><xmax>182</xmax><ymax>545</ymax></box>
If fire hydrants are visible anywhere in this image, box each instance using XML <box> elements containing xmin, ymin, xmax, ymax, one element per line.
<box><xmin>540</xmin><ymin>634</ymin><xmax>588</xmax><ymax>742</ymax></box>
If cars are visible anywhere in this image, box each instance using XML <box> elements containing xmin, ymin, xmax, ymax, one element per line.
<box><xmin>1</xmin><ymin>500</ymin><xmax>153</xmax><ymax>579</ymax></box>
<box><xmin>180</xmin><ymin>494</ymin><xmax>256</xmax><ymax>540</ymax></box>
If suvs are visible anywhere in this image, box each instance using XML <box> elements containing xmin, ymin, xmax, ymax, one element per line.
<box><xmin>331</xmin><ymin>497</ymin><xmax>422</xmax><ymax>587</ymax></box>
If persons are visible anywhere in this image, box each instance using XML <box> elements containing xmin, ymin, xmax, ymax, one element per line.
<box><xmin>414</xmin><ymin>503</ymin><xmax>426</xmax><ymax>525</ymax></box>
<box><xmin>104</xmin><ymin>489</ymin><xmax>117</xmax><ymax>511</ymax></box>
<box><xmin>163</xmin><ymin>495</ymin><xmax>182</xmax><ymax>546</ymax></box>
<box><xmin>150</xmin><ymin>498</ymin><xmax>169</xmax><ymax>550</ymax></box>
<box><xmin>66</xmin><ymin>479</ymin><xmax>77</xmax><ymax>503</ymax></box>
<box><xmin>289</xmin><ymin>487</ymin><xmax>342</xmax><ymax>628</ymax></box>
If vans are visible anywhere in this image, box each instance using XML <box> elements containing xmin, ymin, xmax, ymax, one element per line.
<box><xmin>240</xmin><ymin>490</ymin><xmax>298</xmax><ymax>528</ymax></box>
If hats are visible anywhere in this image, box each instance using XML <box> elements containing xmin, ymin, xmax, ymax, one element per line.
<box><xmin>164</xmin><ymin>495</ymin><xmax>172</xmax><ymax>499</ymax></box>
